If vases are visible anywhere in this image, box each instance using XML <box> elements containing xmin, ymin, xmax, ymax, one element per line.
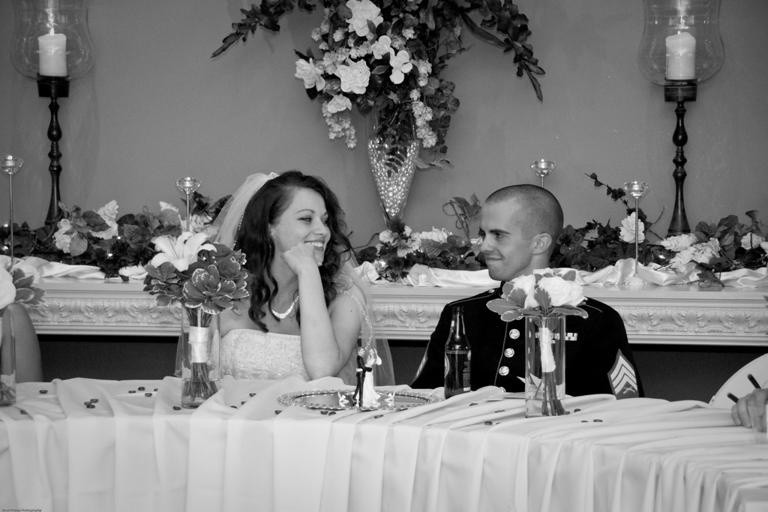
<box><xmin>367</xmin><ymin>120</ymin><xmax>420</xmax><ymax>226</ymax></box>
<box><xmin>522</xmin><ymin>315</ymin><xmax>568</xmax><ymax>420</ymax></box>
<box><xmin>174</xmin><ymin>304</ymin><xmax>218</xmax><ymax>409</ymax></box>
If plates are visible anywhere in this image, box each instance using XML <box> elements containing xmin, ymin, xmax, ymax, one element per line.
<box><xmin>278</xmin><ymin>389</ymin><xmax>436</xmax><ymax>415</ymax></box>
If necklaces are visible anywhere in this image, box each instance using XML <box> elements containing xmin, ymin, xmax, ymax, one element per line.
<box><xmin>262</xmin><ymin>291</ymin><xmax>299</xmax><ymax>320</ymax></box>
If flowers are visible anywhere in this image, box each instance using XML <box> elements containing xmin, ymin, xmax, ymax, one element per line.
<box><xmin>2</xmin><ymin>199</ymin><xmax>767</xmax><ymax>280</ymax></box>
<box><xmin>210</xmin><ymin>0</ymin><xmax>547</xmax><ymax>176</ymax></box>
<box><xmin>139</xmin><ymin>230</ymin><xmax>250</xmax><ymax>402</ymax></box>
<box><xmin>483</xmin><ymin>265</ymin><xmax>605</xmax><ymax>416</ymax></box>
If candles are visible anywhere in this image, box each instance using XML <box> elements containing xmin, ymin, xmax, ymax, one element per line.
<box><xmin>665</xmin><ymin>30</ymin><xmax>697</xmax><ymax>81</ymax></box>
<box><xmin>38</xmin><ymin>27</ymin><xmax>68</xmax><ymax>77</ymax></box>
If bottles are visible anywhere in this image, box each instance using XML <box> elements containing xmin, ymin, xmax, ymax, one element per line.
<box><xmin>444</xmin><ymin>306</ymin><xmax>471</xmax><ymax>400</ymax></box>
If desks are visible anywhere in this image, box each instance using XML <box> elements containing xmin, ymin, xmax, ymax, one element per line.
<box><xmin>0</xmin><ymin>376</ymin><xmax>766</xmax><ymax>511</ymax></box>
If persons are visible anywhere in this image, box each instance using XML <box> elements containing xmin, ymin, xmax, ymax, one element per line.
<box><xmin>175</xmin><ymin>171</ymin><xmax>396</xmax><ymax>387</ymax></box>
<box><xmin>731</xmin><ymin>388</ymin><xmax>768</xmax><ymax>433</ymax></box>
<box><xmin>410</xmin><ymin>184</ymin><xmax>646</xmax><ymax>400</ymax></box>
